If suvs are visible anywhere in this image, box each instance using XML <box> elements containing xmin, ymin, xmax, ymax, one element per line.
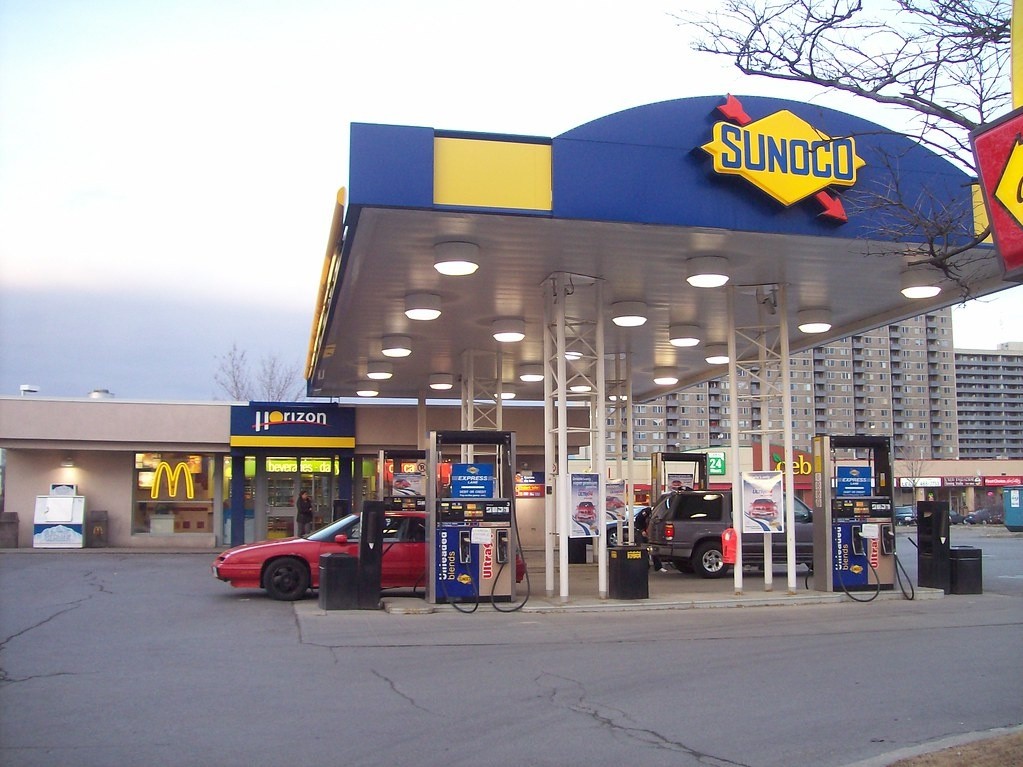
<box><xmin>640</xmin><ymin>488</ymin><xmax>813</xmax><ymax>578</ymax></box>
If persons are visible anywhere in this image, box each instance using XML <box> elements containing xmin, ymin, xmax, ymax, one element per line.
<box><xmin>634</xmin><ymin>508</ymin><xmax>667</xmax><ymax>573</ymax></box>
<box><xmin>296</xmin><ymin>491</ymin><xmax>313</xmax><ymax>538</ymax></box>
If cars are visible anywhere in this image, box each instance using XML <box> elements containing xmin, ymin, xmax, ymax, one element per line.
<box><xmin>208</xmin><ymin>510</ymin><xmax>527</xmax><ymax>601</ymax></box>
<box><xmin>575</xmin><ymin>501</ymin><xmax>597</xmax><ymax>523</ymax></box>
<box><xmin>606</xmin><ymin>503</ymin><xmax>653</xmax><ymax>548</ymax></box>
<box><xmin>894</xmin><ymin>505</ymin><xmax>1004</xmax><ymax>527</ymax></box>
<box><xmin>606</xmin><ymin>496</ymin><xmax>624</xmax><ymax>510</ymax></box>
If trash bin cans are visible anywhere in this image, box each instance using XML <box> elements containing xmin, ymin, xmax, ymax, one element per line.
<box><xmin>0</xmin><ymin>512</ymin><xmax>20</xmax><ymax>548</ymax></box>
<box><xmin>950</xmin><ymin>545</ymin><xmax>983</xmax><ymax>594</ymax></box>
<box><xmin>89</xmin><ymin>510</ymin><xmax>108</xmax><ymax>546</ymax></box>
<box><xmin>319</xmin><ymin>552</ymin><xmax>357</xmax><ymax>609</ymax></box>
